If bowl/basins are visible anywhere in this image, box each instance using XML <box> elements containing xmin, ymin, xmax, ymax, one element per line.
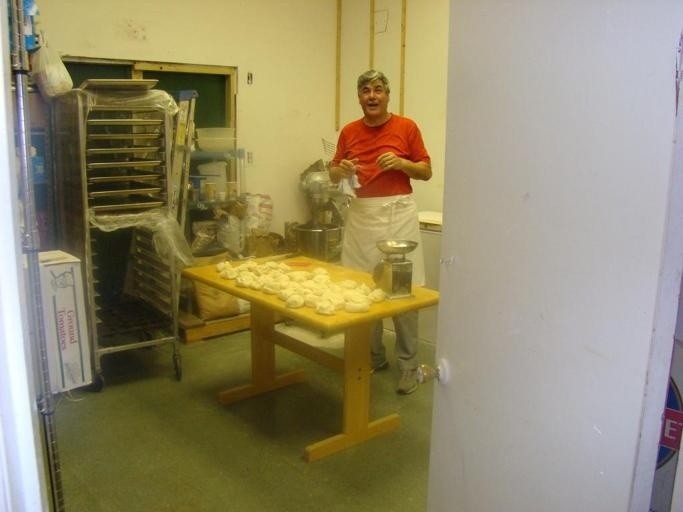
<box><xmin>195</xmin><ymin>127</ymin><xmax>239</xmax><ymax>152</ymax></box>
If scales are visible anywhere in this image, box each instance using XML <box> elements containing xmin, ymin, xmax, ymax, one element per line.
<box><xmin>373</xmin><ymin>239</ymin><xmax>418</xmax><ymax>299</ymax></box>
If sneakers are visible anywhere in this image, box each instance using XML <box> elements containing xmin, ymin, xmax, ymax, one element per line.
<box><xmin>369</xmin><ymin>357</ymin><xmax>389</xmax><ymax>376</ymax></box>
<box><xmin>395</xmin><ymin>364</ymin><xmax>425</xmax><ymax>394</ymax></box>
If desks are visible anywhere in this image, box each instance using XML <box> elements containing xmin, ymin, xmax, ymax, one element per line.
<box><xmin>181</xmin><ymin>250</ymin><xmax>440</xmax><ymax>462</ymax></box>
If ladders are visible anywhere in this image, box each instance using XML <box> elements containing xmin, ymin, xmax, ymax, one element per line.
<box><xmin>121</xmin><ymin>89</ymin><xmax>198</xmax><ymax>317</ymax></box>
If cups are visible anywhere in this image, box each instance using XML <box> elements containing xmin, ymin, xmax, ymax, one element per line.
<box><xmin>215</xmin><ymin>192</ymin><xmax>225</xmax><ymax>200</ymax></box>
<box><xmin>205</xmin><ymin>183</ymin><xmax>216</xmax><ymax>202</ymax></box>
<box><xmin>225</xmin><ymin>181</ymin><xmax>237</xmax><ymax>201</ymax></box>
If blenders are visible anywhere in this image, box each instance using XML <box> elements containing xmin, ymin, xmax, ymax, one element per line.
<box><xmin>291</xmin><ymin>161</ymin><xmax>345</xmax><ymax>264</ymax></box>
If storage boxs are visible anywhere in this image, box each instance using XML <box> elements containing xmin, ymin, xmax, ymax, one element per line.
<box><xmin>26</xmin><ymin>248</ymin><xmax>93</xmax><ymax>398</ymax></box>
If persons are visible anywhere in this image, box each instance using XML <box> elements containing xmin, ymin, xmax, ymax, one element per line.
<box><xmin>328</xmin><ymin>69</ymin><xmax>432</xmax><ymax>393</ymax></box>
